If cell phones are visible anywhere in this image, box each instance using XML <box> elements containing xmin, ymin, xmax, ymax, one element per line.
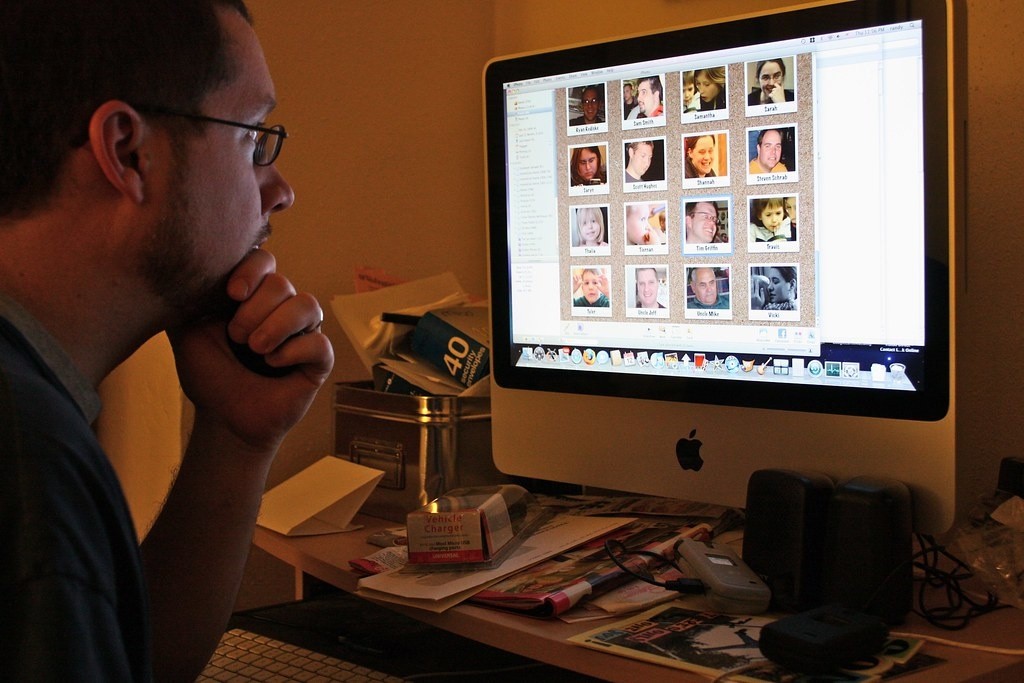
<box><xmin>673</xmin><ymin>538</ymin><xmax>771</xmax><ymax>615</ymax></box>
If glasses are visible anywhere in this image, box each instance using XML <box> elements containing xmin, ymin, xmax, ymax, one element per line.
<box><xmin>69</xmin><ymin>106</ymin><xmax>288</xmax><ymax>166</ymax></box>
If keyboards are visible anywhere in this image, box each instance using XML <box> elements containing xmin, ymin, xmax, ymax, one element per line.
<box><xmin>198</xmin><ymin>628</ymin><xmax>410</xmax><ymax>683</ymax></box>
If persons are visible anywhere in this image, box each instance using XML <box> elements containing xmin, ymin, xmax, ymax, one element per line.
<box><xmin>624</xmin><ymin>76</ymin><xmax>663</xmax><ymax>119</ymax></box>
<box><xmin>688</xmin><ymin>268</ymin><xmax>729</xmax><ymax>308</ymax></box>
<box><xmin>575</xmin><ymin>208</ymin><xmax>608</xmax><ymax>246</ymax></box>
<box><xmin>636</xmin><ymin>268</ymin><xmax>664</xmax><ymax>308</ymax></box>
<box><xmin>683</xmin><ymin>67</ymin><xmax>726</xmax><ymax>114</ymax></box>
<box><xmin>750</xmin><ymin>130</ymin><xmax>789</xmax><ymax>174</ymax></box>
<box><xmin>626</xmin><ymin>205</ymin><xmax>666</xmax><ymax>246</ymax></box>
<box><xmin>751</xmin><ymin>267</ymin><xmax>797</xmax><ymax>311</ymax></box>
<box><xmin>573</xmin><ymin>267</ymin><xmax>609</xmax><ymax>307</ymax></box>
<box><xmin>686</xmin><ymin>203</ymin><xmax>719</xmax><ymax>245</ymax></box>
<box><xmin>570</xmin><ymin>85</ymin><xmax>602</xmax><ymax>127</ymax></box>
<box><xmin>0</xmin><ymin>2</ymin><xmax>334</xmax><ymax>683</ymax></box>
<box><xmin>748</xmin><ymin>58</ymin><xmax>794</xmax><ymax>105</ymax></box>
<box><xmin>750</xmin><ymin>197</ymin><xmax>796</xmax><ymax>242</ymax></box>
<box><xmin>570</xmin><ymin>146</ymin><xmax>607</xmax><ymax>187</ymax></box>
<box><xmin>685</xmin><ymin>135</ymin><xmax>715</xmax><ymax>178</ymax></box>
<box><xmin>625</xmin><ymin>141</ymin><xmax>654</xmax><ymax>181</ymax></box>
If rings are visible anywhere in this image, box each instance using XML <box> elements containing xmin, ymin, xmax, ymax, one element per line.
<box><xmin>304</xmin><ymin>307</ymin><xmax>324</xmax><ymax>335</ymax></box>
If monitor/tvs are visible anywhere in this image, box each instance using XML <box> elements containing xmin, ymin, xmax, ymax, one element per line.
<box><xmin>483</xmin><ymin>0</ymin><xmax>969</xmax><ymax>556</ymax></box>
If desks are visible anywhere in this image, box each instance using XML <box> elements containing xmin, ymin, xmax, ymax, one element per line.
<box><xmin>253</xmin><ymin>505</ymin><xmax>1024</xmax><ymax>683</ymax></box>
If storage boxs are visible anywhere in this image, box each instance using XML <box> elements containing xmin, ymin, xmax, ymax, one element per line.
<box><xmin>332</xmin><ymin>379</ymin><xmax>491</xmax><ymax>524</ymax></box>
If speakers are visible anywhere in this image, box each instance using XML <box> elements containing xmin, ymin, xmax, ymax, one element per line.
<box><xmin>742</xmin><ymin>468</ymin><xmax>914</xmax><ymax>624</ymax></box>
